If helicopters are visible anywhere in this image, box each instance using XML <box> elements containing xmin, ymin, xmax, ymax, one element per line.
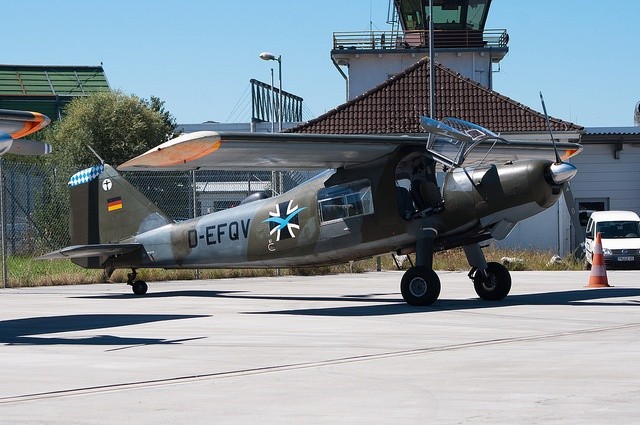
<box><xmin>34</xmin><ymin>91</ymin><xmax>583</xmax><ymax>308</ymax></box>
<box><xmin>1</xmin><ymin>109</ymin><xmax>52</xmax><ymax>156</ymax></box>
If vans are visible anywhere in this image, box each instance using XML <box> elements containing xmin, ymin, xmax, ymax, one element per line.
<box><xmin>583</xmin><ymin>210</ymin><xmax>637</xmax><ymax>270</ymax></box>
